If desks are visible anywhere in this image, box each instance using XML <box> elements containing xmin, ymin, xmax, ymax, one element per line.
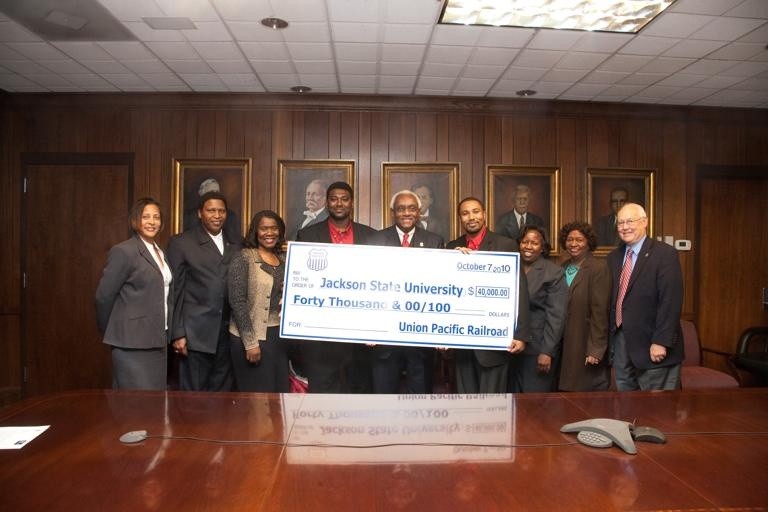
<box><xmin>0</xmin><ymin>387</ymin><xmax>765</xmax><ymax>512</ymax></box>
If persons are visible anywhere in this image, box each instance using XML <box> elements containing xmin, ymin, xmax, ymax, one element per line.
<box><xmin>187</xmin><ymin>179</ymin><xmax>237</xmax><ymax>239</ymax></box>
<box><xmin>510</xmin><ymin>224</ymin><xmax>569</xmax><ymax>392</ymax></box>
<box><xmin>443</xmin><ymin>195</ymin><xmax>530</xmax><ymax>393</ymax></box>
<box><xmin>225</xmin><ymin>210</ymin><xmax>291</xmax><ymax>393</ymax></box>
<box><xmin>495</xmin><ymin>185</ymin><xmax>542</xmax><ymax>241</ymax></box>
<box><xmin>294</xmin><ymin>178</ymin><xmax>330</xmax><ymax>238</ymax></box>
<box><xmin>294</xmin><ymin>181</ymin><xmax>378</xmax><ymax>393</ymax></box>
<box><xmin>555</xmin><ymin>220</ymin><xmax>614</xmax><ymax>391</ymax></box>
<box><xmin>596</xmin><ymin>185</ymin><xmax>628</xmax><ymax>244</ymax></box>
<box><xmin>93</xmin><ymin>196</ymin><xmax>174</xmax><ymax>390</ymax></box>
<box><xmin>408</xmin><ymin>181</ymin><xmax>446</xmax><ymax>240</ymax></box>
<box><xmin>364</xmin><ymin>189</ymin><xmax>450</xmax><ymax>393</ymax></box>
<box><xmin>165</xmin><ymin>190</ymin><xmax>237</xmax><ymax>392</ymax></box>
<box><xmin>605</xmin><ymin>202</ymin><xmax>684</xmax><ymax>391</ymax></box>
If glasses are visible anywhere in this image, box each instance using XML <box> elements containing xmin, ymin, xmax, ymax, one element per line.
<box><xmin>616</xmin><ymin>217</ymin><xmax>642</xmax><ymax>228</ymax></box>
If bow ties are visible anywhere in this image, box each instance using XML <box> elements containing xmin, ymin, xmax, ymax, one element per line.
<box><xmin>417</xmin><ymin>216</ymin><xmax>431</xmax><ymax>225</ymax></box>
<box><xmin>303</xmin><ymin>210</ymin><xmax>316</xmax><ymax>220</ymax></box>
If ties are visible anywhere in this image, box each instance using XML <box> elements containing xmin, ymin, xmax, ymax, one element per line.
<box><xmin>520</xmin><ymin>216</ymin><xmax>524</xmax><ymax>230</ymax></box>
<box><xmin>401</xmin><ymin>234</ymin><xmax>409</xmax><ymax>247</ymax></box>
<box><xmin>615</xmin><ymin>247</ymin><xmax>633</xmax><ymax>328</ymax></box>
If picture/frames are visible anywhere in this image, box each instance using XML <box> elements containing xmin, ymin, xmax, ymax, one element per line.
<box><xmin>483</xmin><ymin>163</ymin><xmax>563</xmax><ymax>257</ymax></box>
<box><xmin>583</xmin><ymin>166</ymin><xmax>656</xmax><ymax>258</ymax></box>
<box><xmin>380</xmin><ymin>161</ymin><xmax>460</xmax><ymax>246</ymax></box>
<box><xmin>276</xmin><ymin>158</ymin><xmax>355</xmax><ymax>252</ymax></box>
<box><xmin>169</xmin><ymin>156</ymin><xmax>251</xmax><ymax>240</ymax></box>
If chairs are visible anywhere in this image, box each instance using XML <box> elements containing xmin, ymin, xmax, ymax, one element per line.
<box><xmin>677</xmin><ymin>319</ymin><xmax>742</xmax><ymax>391</ymax></box>
<box><xmin>733</xmin><ymin>326</ymin><xmax>767</xmax><ymax>385</ymax></box>
